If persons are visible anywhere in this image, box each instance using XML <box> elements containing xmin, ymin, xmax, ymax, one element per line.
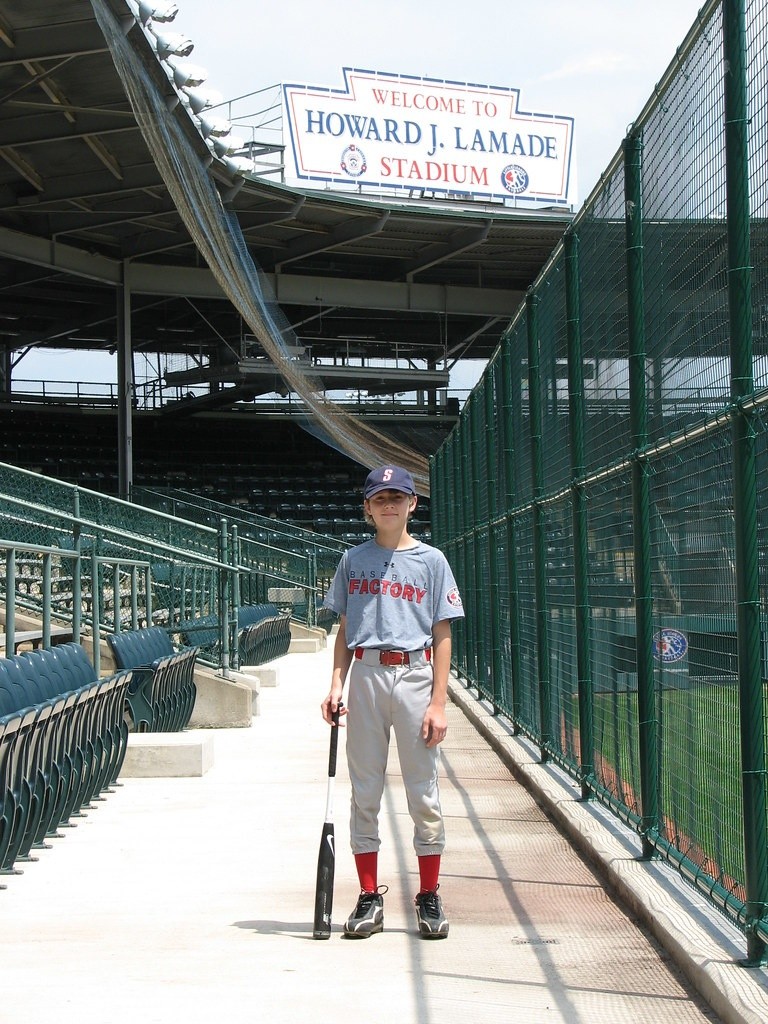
<box><xmin>318</xmin><ymin>464</ymin><xmax>466</xmax><ymax>940</ymax></box>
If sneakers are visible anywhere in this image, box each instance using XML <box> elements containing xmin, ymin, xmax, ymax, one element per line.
<box><xmin>343</xmin><ymin>893</ymin><xmax>384</xmax><ymax>938</ymax></box>
<box><xmin>414</xmin><ymin>894</ymin><xmax>449</xmax><ymax>937</ymax></box>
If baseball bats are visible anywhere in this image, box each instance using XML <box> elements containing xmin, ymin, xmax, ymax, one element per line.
<box><xmin>312</xmin><ymin>702</ymin><xmax>344</xmax><ymax>941</ymax></box>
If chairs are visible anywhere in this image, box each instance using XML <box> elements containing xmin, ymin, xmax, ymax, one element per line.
<box><xmin>0</xmin><ymin>409</ymin><xmax>768</xmax><ymax>890</ymax></box>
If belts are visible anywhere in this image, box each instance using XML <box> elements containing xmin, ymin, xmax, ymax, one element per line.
<box><xmin>356</xmin><ymin>648</ymin><xmax>431</xmax><ymax>665</ymax></box>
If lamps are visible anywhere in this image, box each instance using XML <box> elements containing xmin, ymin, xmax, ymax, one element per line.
<box><xmin>134</xmin><ymin>0</ymin><xmax>241</xmax><ymax>178</ymax></box>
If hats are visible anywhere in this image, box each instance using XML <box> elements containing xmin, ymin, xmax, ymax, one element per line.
<box><xmin>364</xmin><ymin>466</ymin><xmax>415</xmax><ymax>498</ymax></box>
